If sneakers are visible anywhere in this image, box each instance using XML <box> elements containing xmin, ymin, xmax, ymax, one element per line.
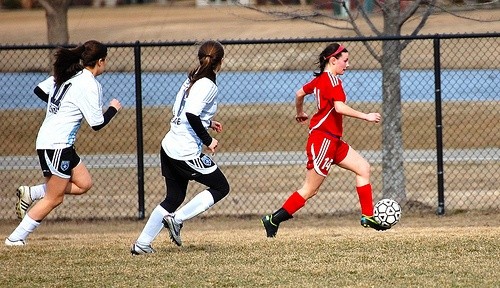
<box><xmin>261</xmin><ymin>215</ymin><xmax>278</xmax><ymax>238</ymax></box>
<box><xmin>16</xmin><ymin>185</ymin><xmax>34</xmax><ymax>222</ymax></box>
<box><xmin>361</xmin><ymin>213</ymin><xmax>388</xmax><ymax>230</ymax></box>
<box><xmin>131</xmin><ymin>243</ymin><xmax>155</xmax><ymax>255</ymax></box>
<box><xmin>161</xmin><ymin>215</ymin><xmax>182</xmax><ymax>247</ymax></box>
<box><xmin>5</xmin><ymin>238</ymin><xmax>24</xmax><ymax>246</ymax></box>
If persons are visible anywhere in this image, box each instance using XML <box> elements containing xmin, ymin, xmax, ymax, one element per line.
<box><xmin>130</xmin><ymin>40</ymin><xmax>230</xmax><ymax>255</ymax></box>
<box><xmin>4</xmin><ymin>40</ymin><xmax>121</xmax><ymax>247</ymax></box>
<box><xmin>261</xmin><ymin>42</ymin><xmax>386</xmax><ymax>238</ymax></box>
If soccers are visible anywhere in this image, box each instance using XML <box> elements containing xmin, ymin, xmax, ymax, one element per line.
<box><xmin>372</xmin><ymin>199</ymin><xmax>403</xmax><ymax>227</ymax></box>
